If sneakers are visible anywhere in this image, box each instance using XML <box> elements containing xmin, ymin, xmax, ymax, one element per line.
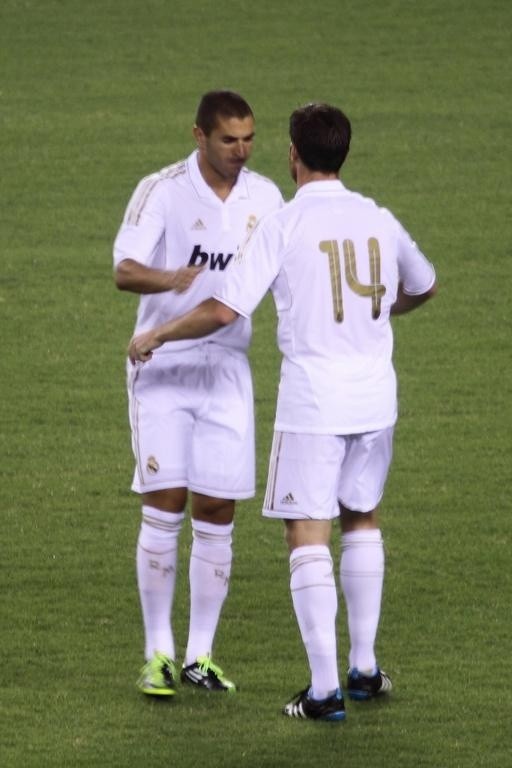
<box><xmin>347</xmin><ymin>665</ymin><xmax>395</xmax><ymax>701</ymax></box>
<box><xmin>180</xmin><ymin>657</ymin><xmax>240</xmax><ymax>695</ymax></box>
<box><xmin>280</xmin><ymin>682</ymin><xmax>347</xmax><ymax>721</ymax></box>
<box><xmin>132</xmin><ymin>649</ymin><xmax>178</xmax><ymax>700</ymax></box>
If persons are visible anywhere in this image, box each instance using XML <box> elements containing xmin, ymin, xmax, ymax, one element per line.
<box><xmin>110</xmin><ymin>87</ymin><xmax>287</xmax><ymax>695</ymax></box>
<box><xmin>126</xmin><ymin>102</ymin><xmax>441</xmax><ymax>724</ymax></box>
